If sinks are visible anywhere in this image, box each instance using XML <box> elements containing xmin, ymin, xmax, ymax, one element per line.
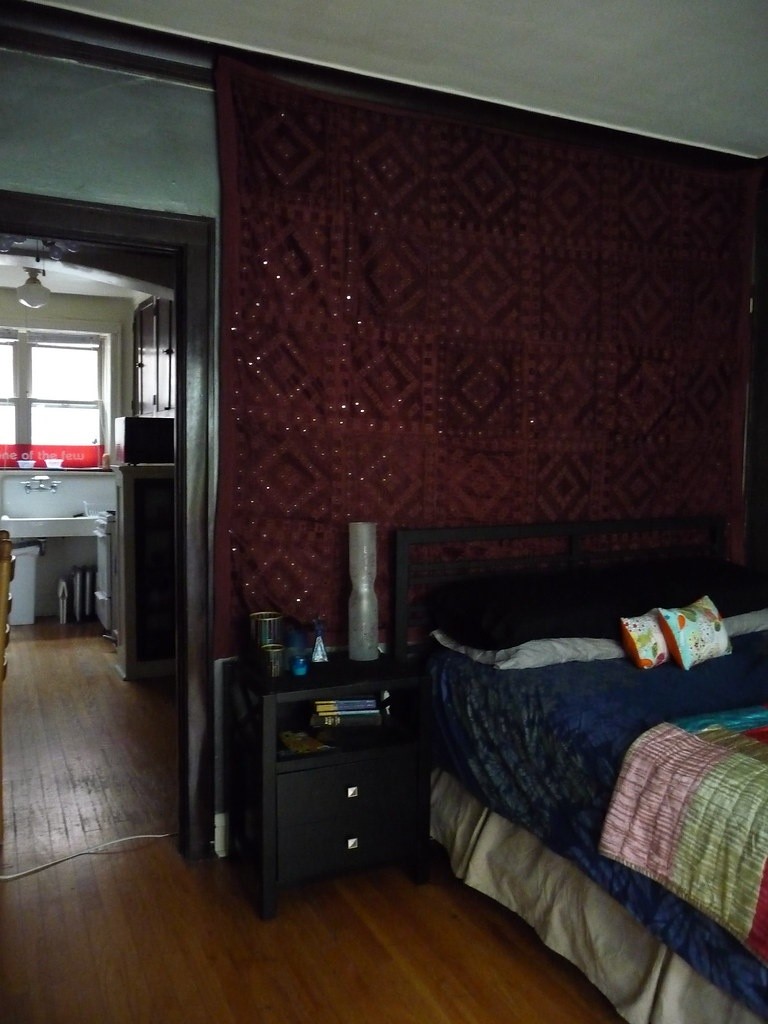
<box><xmin>1</xmin><ymin>511</ymin><xmax>109</xmax><ymax>538</ymax></box>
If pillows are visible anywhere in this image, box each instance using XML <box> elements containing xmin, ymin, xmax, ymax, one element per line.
<box><xmin>587</xmin><ymin>558</ymin><xmax>768</xmax><ymax>640</ymax></box>
<box><xmin>426</xmin><ymin>567</ymin><xmax>596</xmax><ymax>651</ymax></box>
<box><xmin>621</xmin><ymin>606</ymin><xmax>670</xmax><ymax>671</ymax></box>
<box><xmin>658</xmin><ymin>594</ymin><xmax>732</xmax><ymax>670</ymax></box>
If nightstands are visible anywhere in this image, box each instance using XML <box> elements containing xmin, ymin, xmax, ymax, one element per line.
<box><xmin>222</xmin><ymin>650</ymin><xmax>433</xmax><ymax>921</ymax></box>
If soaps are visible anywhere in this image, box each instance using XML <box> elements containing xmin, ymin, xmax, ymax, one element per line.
<box><xmin>35</xmin><ymin>476</ymin><xmax>48</xmax><ymax>479</ymax></box>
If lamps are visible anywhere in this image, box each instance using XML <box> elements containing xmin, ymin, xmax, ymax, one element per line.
<box><xmin>16</xmin><ymin>266</ymin><xmax>51</xmax><ymax>309</ymax></box>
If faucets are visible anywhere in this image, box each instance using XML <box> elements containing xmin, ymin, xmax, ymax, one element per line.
<box><xmin>50</xmin><ymin>480</ymin><xmax>61</xmax><ymax>494</ymax></box>
<box><xmin>20</xmin><ymin>481</ymin><xmax>31</xmax><ymax>494</ymax></box>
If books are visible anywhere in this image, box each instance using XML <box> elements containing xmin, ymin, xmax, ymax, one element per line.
<box><xmin>310</xmin><ymin>701</ymin><xmax>381</xmax><ymax>728</ymax></box>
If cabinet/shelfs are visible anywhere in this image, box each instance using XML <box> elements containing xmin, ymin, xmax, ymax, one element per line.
<box><xmin>133</xmin><ymin>294</ymin><xmax>176</xmax><ymax>418</ymax></box>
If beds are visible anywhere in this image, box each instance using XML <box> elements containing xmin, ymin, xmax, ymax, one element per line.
<box><xmin>390</xmin><ymin>517</ymin><xmax>768</xmax><ymax>1024</ymax></box>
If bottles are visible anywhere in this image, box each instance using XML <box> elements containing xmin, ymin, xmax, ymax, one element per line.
<box><xmin>102</xmin><ymin>454</ymin><xmax>110</xmax><ymax>470</ymax></box>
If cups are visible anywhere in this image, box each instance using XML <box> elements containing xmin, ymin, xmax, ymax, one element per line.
<box><xmin>248</xmin><ymin>612</ymin><xmax>286</xmax><ymax>679</ymax></box>
<box><xmin>291</xmin><ymin>656</ymin><xmax>308</xmax><ymax>676</ymax></box>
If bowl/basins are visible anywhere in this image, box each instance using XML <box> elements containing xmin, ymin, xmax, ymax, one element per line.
<box><xmin>16</xmin><ymin>459</ymin><xmax>64</xmax><ymax>468</ymax></box>
